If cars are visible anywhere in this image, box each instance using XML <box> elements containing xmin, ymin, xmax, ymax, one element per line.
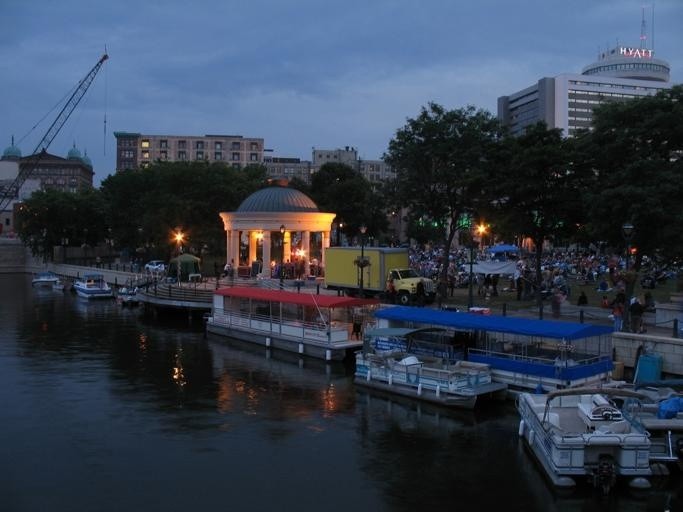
<box><xmin>144</xmin><ymin>260</ymin><xmax>167</xmax><ymax>272</ymax></box>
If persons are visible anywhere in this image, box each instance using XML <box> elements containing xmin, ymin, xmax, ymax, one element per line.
<box><xmin>294</xmin><ymin>258</ymin><xmax>301</xmax><ymax>280</ymax></box>
<box><xmin>94</xmin><ymin>254</ymin><xmax>100</xmax><ymax>268</ymax></box>
<box><xmin>409</xmin><ymin>243</ymin><xmax>682</xmax><ymax>333</ymax></box>
<box><xmin>310</xmin><ymin>257</ymin><xmax>318</xmax><ymax>276</ymax></box>
<box><xmin>284</xmin><ymin>258</ymin><xmax>292</xmax><ymax>280</ymax></box>
<box><xmin>221</xmin><ymin>262</ymin><xmax>230</xmax><ymax>278</ymax></box>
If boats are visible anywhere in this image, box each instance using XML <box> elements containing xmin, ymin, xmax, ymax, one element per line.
<box><xmin>29</xmin><ymin>271</ymin><xmax>65</xmax><ymax>291</ymax></box>
<box><xmin>371</xmin><ymin>307</ymin><xmax>615</xmax><ymax>396</ymax></box>
<box><xmin>203</xmin><ymin>286</ymin><xmax>380</xmax><ymax>362</ymax></box>
<box><xmin>353</xmin><ymin>348</ymin><xmax>510</xmax><ymax>409</ymax></box>
<box><xmin>73</xmin><ymin>272</ymin><xmax>113</xmax><ymax>300</ymax></box>
<box><xmin>514</xmin><ymin>387</ymin><xmax>652</xmax><ymax>495</ymax></box>
<box><xmin>570</xmin><ymin>306</ymin><xmax>683</xmax><ymax>464</ymax></box>
<box><xmin>118</xmin><ymin>284</ymin><xmax>143</xmax><ymax>304</ymax></box>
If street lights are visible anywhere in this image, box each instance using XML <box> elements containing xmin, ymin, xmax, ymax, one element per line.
<box><xmin>175</xmin><ymin>224</ymin><xmax>183</xmax><ymax>287</ymax></box>
<box><xmin>297</xmin><ymin>250</ymin><xmax>304</xmax><ymax>293</ymax></box>
<box><xmin>467</xmin><ymin>224</ymin><xmax>478</xmax><ymax>310</ymax></box>
<box><xmin>622</xmin><ymin>221</ymin><xmax>637</xmax><ymax>271</ymax></box>
<box><xmin>358</xmin><ymin>221</ymin><xmax>368</xmax><ymax>299</ymax></box>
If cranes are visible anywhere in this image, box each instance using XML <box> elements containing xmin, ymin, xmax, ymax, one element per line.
<box><xmin>0</xmin><ymin>43</ymin><xmax>108</xmax><ymax>216</ymax></box>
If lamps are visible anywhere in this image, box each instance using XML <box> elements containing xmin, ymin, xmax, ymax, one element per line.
<box><xmin>280</xmin><ymin>224</ymin><xmax>286</xmax><ymax>235</ymax></box>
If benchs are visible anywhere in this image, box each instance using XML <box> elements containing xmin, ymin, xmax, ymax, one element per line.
<box><xmin>576</xmin><ymin>279</ymin><xmax>596</xmax><ymax>285</ymax></box>
<box><xmin>576</xmin><ymin>275</ymin><xmax>591</xmax><ymax>280</ymax></box>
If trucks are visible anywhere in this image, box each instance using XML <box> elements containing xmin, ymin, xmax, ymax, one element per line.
<box><xmin>325</xmin><ymin>247</ymin><xmax>436</xmax><ymax>306</ymax></box>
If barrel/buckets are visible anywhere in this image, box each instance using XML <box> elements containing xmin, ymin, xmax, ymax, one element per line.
<box><xmin>612</xmin><ymin>362</ymin><xmax>624</xmax><ymax>379</ymax></box>
<box><xmin>637</xmin><ymin>354</ymin><xmax>663</xmax><ymax>385</ymax></box>
